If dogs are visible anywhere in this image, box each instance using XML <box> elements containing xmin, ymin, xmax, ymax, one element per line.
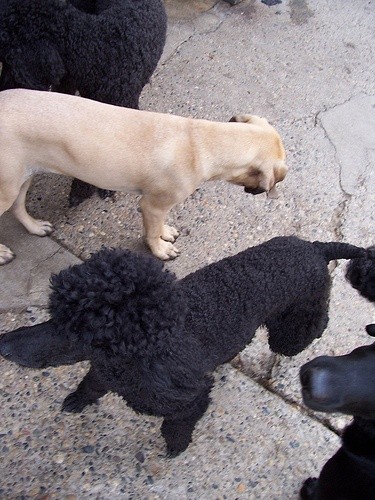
<box><xmin>0</xmin><ymin>0</ymin><xmax>168</xmax><ymax>207</ymax></box>
<box><xmin>0</xmin><ymin>233</ymin><xmax>375</xmax><ymax>459</ymax></box>
<box><xmin>298</xmin><ymin>323</ymin><xmax>375</xmax><ymax>500</ymax></box>
<box><xmin>0</xmin><ymin>87</ymin><xmax>288</xmax><ymax>266</ymax></box>
